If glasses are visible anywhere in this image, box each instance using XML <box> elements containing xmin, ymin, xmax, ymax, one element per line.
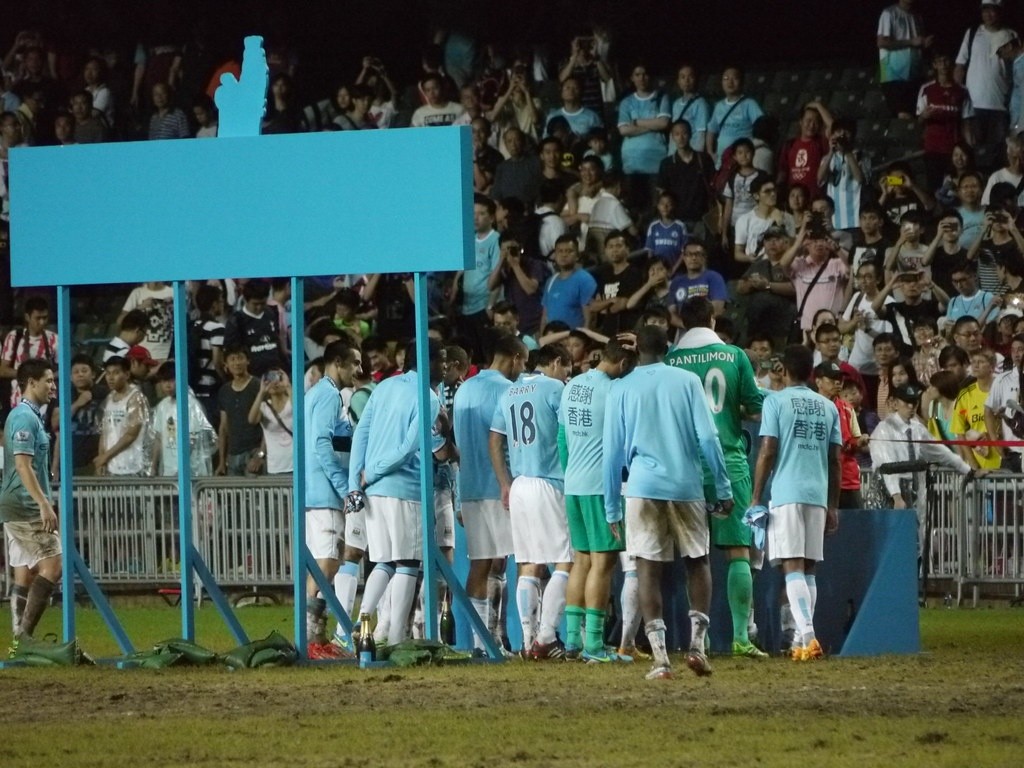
<box><xmin>953</xmin><ymin>332</ymin><xmax>981</xmax><ymax>339</ymax></box>
<box><xmin>760</xmin><ymin>188</ymin><xmax>776</xmax><ymax>195</ymax></box>
<box><xmin>819</xmin><ymin>337</ymin><xmax>840</xmax><ymax>344</ymax></box>
<box><xmin>721</xmin><ymin>76</ymin><xmax>738</xmax><ymax>80</ymax></box>
<box><xmin>856</xmin><ymin>273</ymin><xmax>873</xmax><ymax>281</ymax></box>
<box><xmin>950</xmin><ymin>275</ymin><xmax>973</xmax><ymax>286</ymax></box>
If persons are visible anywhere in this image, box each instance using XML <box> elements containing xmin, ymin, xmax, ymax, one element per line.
<box><xmin>452</xmin><ymin>335</ymin><xmax>528</xmax><ymax>657</ymax></box>
<box><xmin>618</xmin><ymin>551</ymin><xmax>652</xmax><ymax>663</ymax></box>
<box><xmin>814</xmin><ymin>362</ymin><xmax>871</xmax><ymax>507</ymax></box>
<box><xmin>558</xmin><ymin>333</ymin><xmax>637</xmax><ymax>666</ymax></box>
<box><xmin>867</xmin><ymin>385</ymin><xmax>990</xmax><ymax>511</ymax></box>
<box><xmin>602</xmin><ymin>325</ymin><xmax>735</xmax><ymax>679</ymax></box>
<box><xmin>0</xmin><ymin>1</ymin><xmax>1024</xmax><ymax>574</ymax></box>
<box><xmin>663</xmin><ymin>296</ymin><xmax>768</xmax><ymax>661</ymax></box>
<box><xmin>748</xmin><ymin>345</ymin><xmax>843</xmax><ymax>659</ymax></box>
<box><xmin>304</xmin><ymin>338</ymin><xmax>469</xmax><ymax>661</ymax></box>
<box><xmin>490</xmin><ymin>343</ymin><xmax>573</xmax><ymax>661</ymax></box>
<box><xmin>0</xmin><ymin>359</ymin><xmax>61</xmax><ymax>657</ymax></box>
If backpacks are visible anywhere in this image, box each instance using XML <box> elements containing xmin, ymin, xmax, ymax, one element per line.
<box><xmin>185</xmin><ymin>316</ymin><xmax>219</xmax><ymax>385</ymax></box>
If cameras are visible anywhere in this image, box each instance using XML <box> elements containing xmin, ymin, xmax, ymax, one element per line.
<box><xmin>924</xmin><ymin>338</ymin><xmax>936</xmax><ymax>346</ymax></box>
<box><xmin>760</xmin><ymin>361</ymin><xmax>776</xmax><ymax>369</ymax></box>
<box><xmin>832</xmin><ymin>136</ymin><xmax>849</xmax><ymax>150</ymax></box>
<box><xmin>805</xmin><ymin>211</ymin><xmax>826</xmax><ymax>239</ymax></box>
<box><xmin>507</xmin><ymin>246</ymin><xmax>518</xmax><ymax>257</ymax></box>
<box><xmin>267</xmin><ymin>370</ymin><xmax>279</xmax><ymax>382</ymax></box>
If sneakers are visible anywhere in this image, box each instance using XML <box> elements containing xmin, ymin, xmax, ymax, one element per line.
<box><xmin>731</xmin><ymin>641</ymin><xmax>769</xmax><ymax>661</ymax></box>
<box><xmin>498</xmin><ymin>645</ymin><xmax>518</xmax><ymax>659</ymax></box>
<box><xmin>644</xmin><ymin>662</ymin><xmax>673</xmax><ymax>681</ymax></box>
<box><xmin>801</xmin><ymin>640</ymin><xmax>824</xmax><ymax>662</ymax></box>
<box><xmin>687</xmin><ymin>652</ymin><xmax>712</xmax><ymax>678</ymax></box>
<box><xmin>617</xmin><ymin>648</ymin><xmax>653</xmax><ymax>660</ymax></box>
<box><xmin>576</xmin><ymin>646</ymin><xmax>636</xmax><ymax>668</ymax></box>
<box><xmin>308</xmin><ymin>639</ymin><xmax>356</xmax><ymax>663</ymax></box>
<box><xmin>563</xmin><ymin>645</ymin><xmax>584</xmax><ymax>662</ymax></box>
<box><xmin>527</xmin><ymin>632</ymin><xmax>565</xmax><ymax>662</ymax></box>
<box><xmin>790</xmin><ymin>647</ymin><xmax>803</xmax><ymax>661</ymax></box>
<box><xmin>472</xmin><ymin>648</ymin><xmax>488</xmax><ymax>660</ymax></box>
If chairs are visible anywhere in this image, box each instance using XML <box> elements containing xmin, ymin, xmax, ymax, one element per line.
<box><xmin>611</xmin><ymin>55</ymin><xmax>924</xmax><ymax>189</ymax></box>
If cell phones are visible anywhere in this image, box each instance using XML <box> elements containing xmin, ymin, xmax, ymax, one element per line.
<box><xmin>887</xmin><ymin>177</ymin><xmax>902</xmax><ymax>185</ymax></box>
<box><xmin>579</xmin><ymin>39</ymin><xmax>594</xmax><ymax>48</ymax></box>
<box><xmin>750</xmin><ymin>272</ymin><xmax>760</xmax><ymax>278</ymax></box>
<box><xmin>991</xmin><ymin>214</ymin><xmax>1007</xmax><ymax>223</ymax></box>
<box><xmin>999</xmin><ymin>294</ymin><xmax>1019</xmax><ymax>304</ymax></box>
<box><xmin>944</xmin><ymin>223</ymin><xmax>958</xmax><ymax>231</ymax></box>
<box><xmin>900</xmin><ymin>274</ymin><xmax>918</xmax><ymax>282</ymax></box>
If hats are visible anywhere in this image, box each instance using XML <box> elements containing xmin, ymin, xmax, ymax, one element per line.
<box><xmin>891</xmin><ymin>385</ymin><xmax>921</xmax><ymax>402</ymax></box>
<box><xmin>761</xmin><ymin>225</ymin><xmax>788</xmax><ymax>238</ymax></box>
<box><xmin>813</xmin><ymin>360</ymin><xmax>851</xmax><ymax>378</ymax></box>
<box><xmin>128</xmin><ymin>346</ymin><xmax>159</xmax><ymax>366</ymax></box>
